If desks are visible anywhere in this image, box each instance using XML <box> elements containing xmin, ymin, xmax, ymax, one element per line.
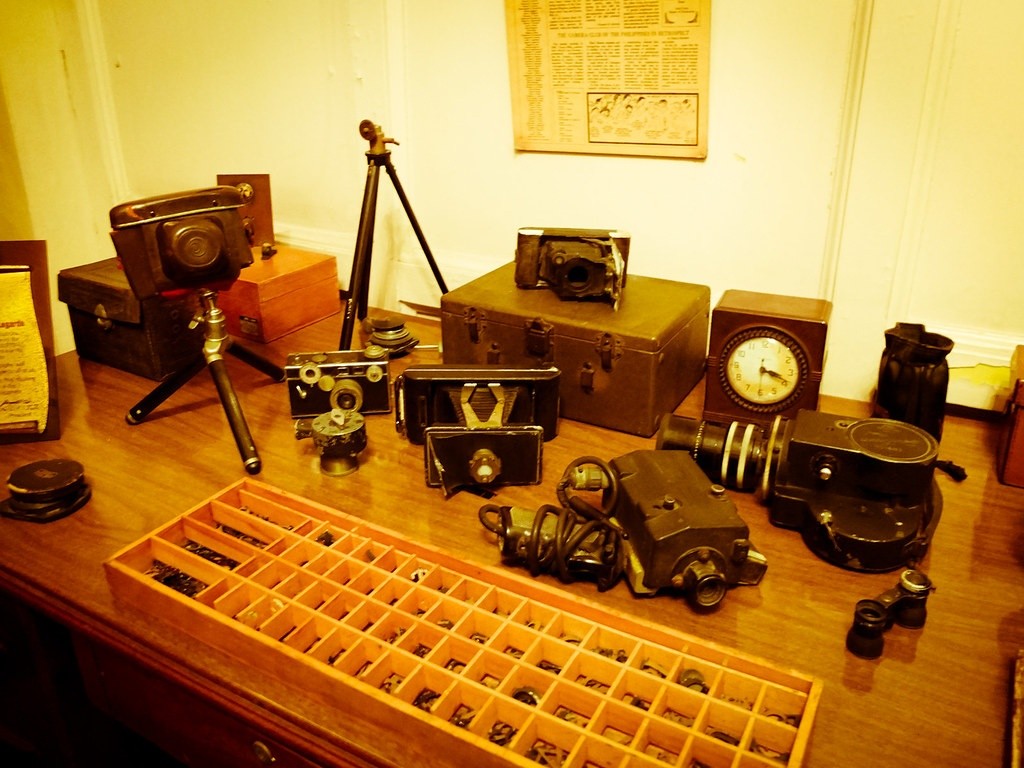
<box><xmin>0</xmin><ymin>278</ymin><xmax>1022</xmax><ymax>767</ymax></box>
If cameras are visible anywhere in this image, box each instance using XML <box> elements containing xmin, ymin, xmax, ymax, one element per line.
<box><xmin>108</xmin><ymin>183</ymin><xmax>253</xmax><ymax>297</ymax></box>
<box><xmin>284</xmin><ymin>348</ymin><xmax>392</xmax><ymax>419</ymax></box>
<box><xmin>423</xmin><ymin>422</ymin><xmax>542</xmax><ymax>491</ymax></box>
<box><xmin>393</xmin><ymin>360</ymin><xmax>563</xmax><ymax>443</ymax></box>
<box><xmin>568</xmin><ymin>447</ymin><xmax>768</xmax><ymax>606</ymax></box>
<box><xmin>512</xmin><ymin>227</ymin><xmax>631</xmax><ymax>302</ymax></box>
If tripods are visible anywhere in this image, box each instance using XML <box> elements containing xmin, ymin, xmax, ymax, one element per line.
<box><xmin>335</xmin><ymin>118</ymin><xmax>448</xmax><ymax>349</ymax></box>
<box><xmin>125</xmin><ymin>288</ymin><xmax>286</xmax><ymax>473</ymax></box>
